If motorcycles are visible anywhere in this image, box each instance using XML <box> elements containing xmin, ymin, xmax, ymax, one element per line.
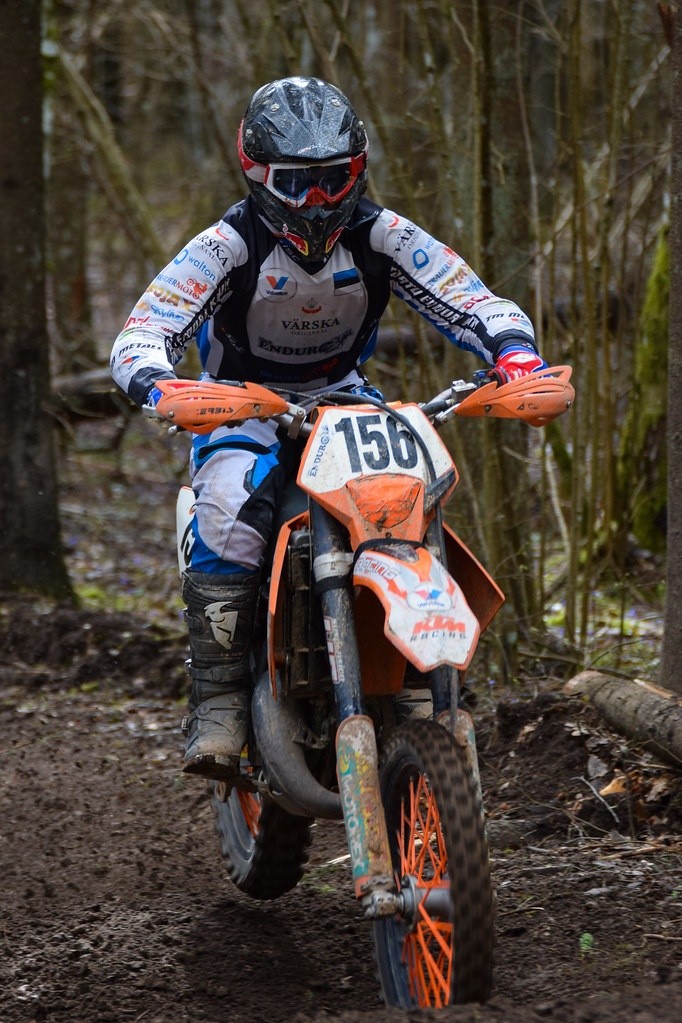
<box><xmin>149</xmin><ymin>365</ymin><xmax>573</xmax><ymax>1009</ymax></box>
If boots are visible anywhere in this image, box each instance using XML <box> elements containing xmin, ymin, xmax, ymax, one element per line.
<box><xmin>180</xmin><ymin>569</ymin><xmax>258</xmax><ymax>773</ymax></box>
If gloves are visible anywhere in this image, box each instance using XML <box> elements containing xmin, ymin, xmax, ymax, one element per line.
<box><xmin>144</xmin><ymin>377</ymin><xmax>192</xmax><ymax>407</ymax></box>
<box><xmin>489</xmin><ymin>346</ymin><xmax>555</xmax><ymax>389</ymax></box>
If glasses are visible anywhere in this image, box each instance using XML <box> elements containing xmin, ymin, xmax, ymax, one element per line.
<box><xmin>263</xmin><ymin>157</ymin><xmax>357</xmax><ymax>209</ymax></box>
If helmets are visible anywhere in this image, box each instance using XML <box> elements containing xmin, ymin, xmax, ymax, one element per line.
<box><xmin>238</xmin><ymin>77</ymin><xmax>368</xmax><ymax>276</ymax></box>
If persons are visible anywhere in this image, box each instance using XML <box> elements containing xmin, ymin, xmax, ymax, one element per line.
<box><xmin>109</xmin><ymin>77</ymin><xmax>552</xmax><ymax>772</ymax></box>
